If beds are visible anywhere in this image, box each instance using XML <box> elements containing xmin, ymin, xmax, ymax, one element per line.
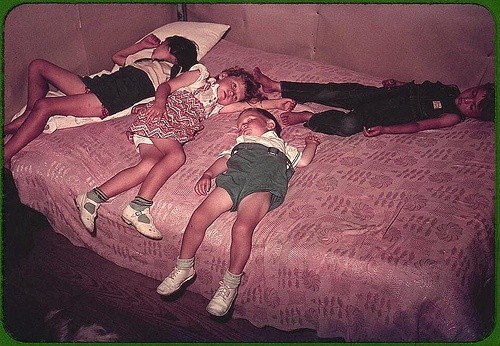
<box><xmin>9</xmin><ymin>37</ymin><xmax>496</xmax><ymax>343</ymax></box>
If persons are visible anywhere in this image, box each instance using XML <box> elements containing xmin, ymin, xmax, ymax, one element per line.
<box><xmin>3</xmin><ymin>34</ymin><xmax>198</xmax><ymax>168</ymax></box>
<box><xmin>251</xmin><ymin>67</ymin><xmax>495</xmax><ymax>139</ymax></box>
<box><xmin>156</xmin><ymin>109</ymin><xmax>323</xmax><ymax>317</ymax></box>
<box><xmin>72</xmin><ymin>61</ymin><xmax>299</xmax><ymax>241</ymax></box>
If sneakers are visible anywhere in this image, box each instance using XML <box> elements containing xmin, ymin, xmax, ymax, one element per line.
<box><xmin>206</xmin><ymin>281</ymin><xmax>238</xmax><ymax>316</ymax></box>
<box><xmin>156</xmin><ymin>266</ymin><xmax>196</xmax><ymax>297</ymax></box>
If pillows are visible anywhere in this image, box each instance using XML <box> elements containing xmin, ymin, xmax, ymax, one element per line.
<box><xmin>129</xmin><ymin>21</ymin><xmax>231</xmax><ymax>63</ymax></box>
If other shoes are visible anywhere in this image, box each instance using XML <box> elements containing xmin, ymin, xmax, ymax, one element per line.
<box><xmin>75</xmin><ymin>192</ymin><xmax>101</xmax><ymax>233</ymax></box>
<box><xmin>122</xmin><ymin>204</ymin><xmax>164</xmax><ymax>240</ymax></box>
